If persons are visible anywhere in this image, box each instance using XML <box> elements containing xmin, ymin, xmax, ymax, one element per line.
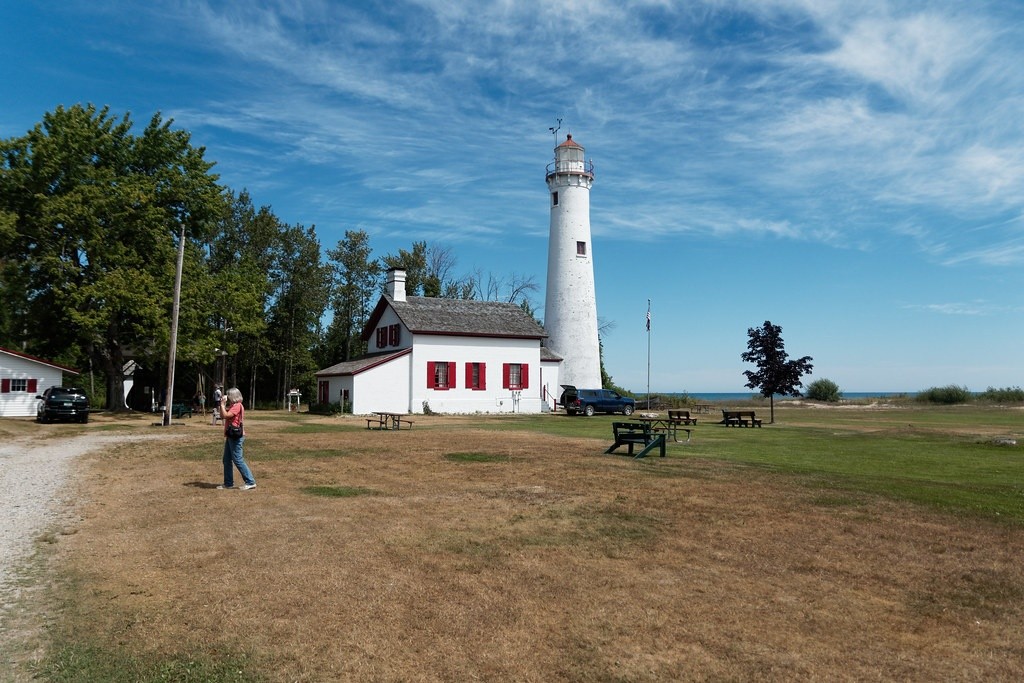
<box><xmin>216</xmin><ymin>388</ymin><xmax>257</xmax><ymax>490</ymax></box>
<box><xmin>193</xmin><ymin>384</ymin><xmax>224</xmax><ymax>415</ymax></box>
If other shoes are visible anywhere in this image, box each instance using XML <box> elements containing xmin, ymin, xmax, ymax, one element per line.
<box><xmin>216</xmin><ymin>485</ymin><xmax>236</xmax><ymax>489</ymax></box>
<box><xmin>239</xmin><ymin>485</ymin><xmax>257</xmax><ymax>491</ymax></box>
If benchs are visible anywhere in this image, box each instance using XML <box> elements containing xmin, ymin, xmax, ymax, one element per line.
<box><xmin>171</xmin><ymin>402</ymin><xmax>192</xmax><ymax>418</ymax></box>
<box><xmin>394</xmin><ymin>419</ymin><xmax>415</xmax><ymax>423</ymax></box>
<box><xmin>668</xmin><ymin>411</ymin><xmax>697</xmax><ymax>426</ymax></box>
<box><xmin>721</xmin><ymin>409</ymin><xmax>763</xmax><ymax>428</ymax></box>
<box><xmin>366</xmin><ymin>419</ymin><xmax>387</xmax><ymax>424</ymax></box>
<box><xmin>650</xmin><ymin>427</ymin><xmax>694</xmax><ymax>433</ymax></box>
<box><xmin>603</xmin><ymin>421</ymin><xmax>668</xmax><ymax>460</ymax></box>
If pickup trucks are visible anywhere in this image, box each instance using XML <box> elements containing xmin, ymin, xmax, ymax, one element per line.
<box><xmin>560</xmin><ymin>384</ymin><xmax>636</xmax><ymax>417</ymax></box>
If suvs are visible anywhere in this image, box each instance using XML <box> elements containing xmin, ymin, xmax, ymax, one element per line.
<box><xmin>35</xmin><ymin>385</ymin><xmax>91</xmax><ymax>425</ymax></box>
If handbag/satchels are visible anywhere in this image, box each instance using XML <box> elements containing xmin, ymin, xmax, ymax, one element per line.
<box><xmin>225</xmin><ymin>423</ymin><xmax>244</xmax><ymax>439</ymax></box>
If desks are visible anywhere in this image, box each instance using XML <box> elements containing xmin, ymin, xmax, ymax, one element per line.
<box><xmin>629</xmin><ymin>418</ymin><xmax>690</xmax><ymax>444</ymax></box>
<box><xmin>367</xmin><ymin>412</ymin><xmax>412</xmax><ymax>431</ymax></box>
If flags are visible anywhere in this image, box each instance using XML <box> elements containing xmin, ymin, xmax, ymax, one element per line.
<box><xmin>645</xmin><ymin>299</ymin><xmax>651</xmax><ymax>331</ymax></box>
